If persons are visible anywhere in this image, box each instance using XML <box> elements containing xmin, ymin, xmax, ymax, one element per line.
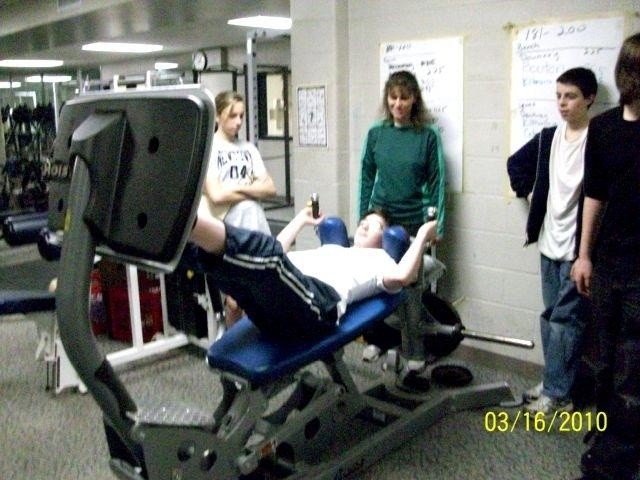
<box><xmin>506</xmin><ymin>68</ymin><xmax>597</xmax><ymax>421</ymax></box>
<box><xmin>570</xmin><ymin>33</ymin><xmax>640</xmax><ymax>480</ymax></box>
<box><xmin>182</xmin><ymin>205</ymin><xmax>438</xmax><ymax>350</ymax></box>
<box><xmin>358</xmin><ymin>72</ymin><xmax>447</xmax><ymax>376</ymax></box>
<box><xmin>202</xmin><ymin>90</ymin><xmax>277</xmax><ymax>331</ymax></box>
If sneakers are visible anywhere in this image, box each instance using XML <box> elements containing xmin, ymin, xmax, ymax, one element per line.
<box><xmin>523</xmin><ymin>394</ymin><xmax>574</xmax><ymax>420</ymax></box>
<box><xmin>522</xmin><ymin>381</ymin><xmax>544</xmax><ymax>403</ymax></box>
<box><xmin>406</xmin><ymin>360</ymin><xmax>425</xmax><ymax>374</ymax></box>
<box><xmin>361</xmin><ymin>344</ymin><xmax>381</xmax><ymax>363</ymax></box>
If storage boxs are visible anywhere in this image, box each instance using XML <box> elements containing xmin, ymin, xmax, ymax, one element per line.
<box><xmin>108</xmin><ymin>276</ymin><xmax>162</xmax><ymax>344</ymax></box>
